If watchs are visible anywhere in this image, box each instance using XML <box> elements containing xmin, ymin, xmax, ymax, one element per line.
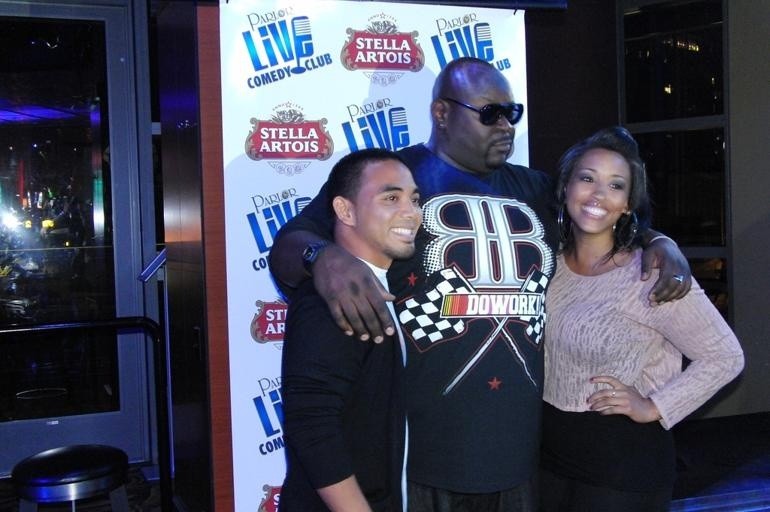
<box><xmin>301</xmin><ymin>238</ymin><xmax>333</xmax><ymax>279</ymax></box>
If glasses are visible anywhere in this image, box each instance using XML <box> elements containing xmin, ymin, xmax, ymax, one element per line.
<box><xmin>436</xmin><ymin>96</ymin><xmax>523</xmax><ymax>125</ymax></box>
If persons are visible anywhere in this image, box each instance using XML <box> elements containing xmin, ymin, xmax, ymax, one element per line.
<box><xmin>539</xmin><ymin>126</ymin><xmax>746</xmax><ymax>512</ymax></box>
<box><xmin>277</xmin><ymin>147</ymin><xmax>424</xmax><ymax>512</ymax></box>
<box><xmin>268</xmin><ymin>56</ymin><xmax>692</xmax><ymax>512</ymax></box>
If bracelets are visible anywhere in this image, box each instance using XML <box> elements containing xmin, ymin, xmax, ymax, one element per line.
<box><xmin>645</xmin><ymin>235</ymin><xmax>678</xmax><ymax>247</ymax></box>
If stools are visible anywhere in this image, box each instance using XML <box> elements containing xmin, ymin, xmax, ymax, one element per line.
<box><xmin>8</xmin><ymin>445</ymin><xmax>133</xmax><ymax>511</ymax></box>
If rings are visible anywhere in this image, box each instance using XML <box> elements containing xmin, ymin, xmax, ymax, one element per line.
<box><xmin>671</xmin><ymin>273</ymin><xmax>685</xmax><ymax>283</ymax></box>
<box><xmin>610</xmin><ymin>389</ymin><xmax>616</xmax><ymax>398</ymax></box>
